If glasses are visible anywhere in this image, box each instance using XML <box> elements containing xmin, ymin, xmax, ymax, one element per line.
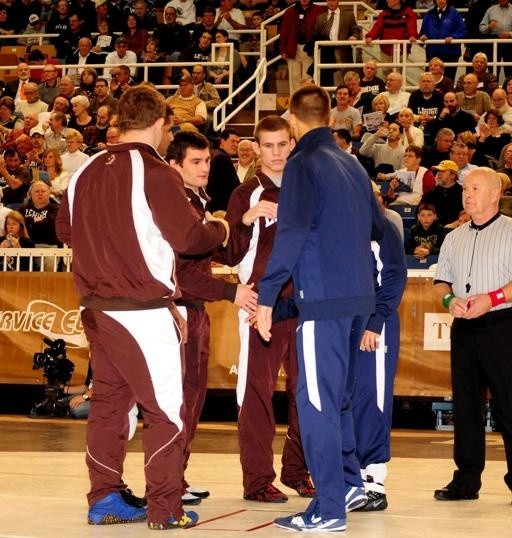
<box><xmin>23</xmin><ymin>91</ymin><xmax>36</xmax><ymax>96</ymax></box>
<box><xmin>237</xmin><ymin>147</ymin><xmax>255</xmax><ymax>153</ymax></box>
<box><xmin>58</xmin><ymin>4</ymin><xmax>68</xmax><ymax>9</ymax></box>
<box><xmin>404</xmin><ymin>154</ymin><xmax>417</xmax><ymax>158</ymax></box>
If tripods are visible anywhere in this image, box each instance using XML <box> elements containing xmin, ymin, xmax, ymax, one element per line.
<box><xmin>29</xmin><ymin>385</ymin><xmax>69</xmax><ymax>419</ymax></box>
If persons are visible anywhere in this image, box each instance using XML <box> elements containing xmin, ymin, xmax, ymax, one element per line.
<box><xmin>110</xmin><ymin>67</ymin><xmax>139</xmax><ymax>99</ymax></box>
<box><xmin>163</xmin><ymin>1</ymin><xmax>197</xmax><ymax>28</ymax></box>
<box><xmin>24</xmin><ymin>127</ymin><xmax>47</xmax><ymax>159</ymax></box>
<box><xmin>428</xmin><ymin>91</ymin><xmax>477</xmax><ymax>135</ymax></box>
<box><xmin>54</xmin><ymin>75</ymin><xmax>87</xmax><ymax>100</ymax></box>
<box><xmin>1</xmin><ymin>63</ymin><xmax>40</xmax><ymax>101</ymax></box>
<box><xmin>1</xmin><ymin>148</ymin><xmax>29</xmax><ymax>211</ymax></box>
<box><xmin>127</xmin><ymin>2</ymin><xmax>157</xmax><ymax>32</ymax></box>
<box><xmin>240</xmin><ymin>12</ymin><xmax>268</xmax><ymax>67</ymax></box>
<box><xmin>103</xmin><ymin>128</ymin><xmax>118</xmax><ymax>149</ymax></box>
<box><xmin>252</xmin><ymin>85</ymin><xmax>383</xmax><ymax>532</ymax></box>
<box><xmin>193</xmin><ymin>8</ymin><xmax>221</xmax><ymax>52</ymax></box>
<box><xmin>365</xmin><ymin>0</ymin><xmax>419</xmax><ymax>77</ymax></box>
<box><xmin>416</xmin><ymin>0</ymin><xmax>467</xmax><ymax>82</ymax></box>
<box><xmin>35</xmin><ymin>65</ymin><xmax>62</xmax><ymax>109</ymax></box>
<box><xmin>163</xmin><ymin>79</ymin><xmax>207</xmax><ymax>132</ymax></box>
<box><xmin>229</xmin><ymin>116</ymin><xmax>316</xmax><ymax>503</ymax></box>
<box><xmin>213</xmin><ymin>0</ymin><xmax>248</xmax><ymax>50</ymax></box>
<box><xmin>38</xmin><ymin>97</ymin><xmax>71</xmax><ymax>126</ymax></box>
<box><xmin>457</xmin><ymin>53</ymin><xmax>499</xmax><ymax>94</ymax></box>
<box><xmin>242</xmin><ymin>216</ymin><xmax>409</xmax><ymax>512</ymax></box>
<box><xmin>73</xmin><ymin>70</ymin><xmax>98</xmax><ymax>100</ymax></box>
<box><xmin>205</xmin><ymin>29</ymin><xmax>241</xmax><ymax>96</ymax></box>
<box><xmin>0</xmin><ymin>97</ymin><xmax>24</xmax><ymax>142</ymax></box>
<box><xmin>176</xmin><ymin>32</ymin><xmax>213</xmax><ymax>81</ymax></box>
<box><xmin>479</xmin><ymin>89</ymin><xmax>511</xmax><ymax>133</ymax></box>
<box><xmin>152</xmin><ymin>8</ymin><xmax>193</xmax><ymax>64</ymax></box>
<box><xmin>24</xmin><ymin>13</ymin><xmax>46</xmax><ymax>44</ymax></box>
<box><xmin>65</xmin><ymin>37</ymin><xmax>105</xmax><ymax>76</ymax></box>
<box><xmin>409</xmin><ymin>73</ymin><xmax>445</xmax><ymax>128</ymax></box>
<box><xmin>83</xmin><ymin>106</ymin><xmax>114</xmax><ymax>151</ymax></box>
<box><xmin>28</xmin><ymin>49</ymin><xmax>61</xmax><ymax>77</ymax></box>
<box><xmin>3</xmin><ymin>111</ymin><xmax>40</xmax><ymax>155</ymax></box>
<box><xmin>130</xmin><ymin>37</ymin><xmax>167</xmax><ymax>85</ymax></box>
<box><xmin>56</xmin><ymin>85</ymin><xmax>230</xmax><ymax>530</ymax></box>
<box><xmin>497</xmin><ymin>143</ymin><xmax>512</xmax><ymax>197</ymax></box>
<box><xmin>377</xmin><ymin>146</ymin><xmax>427</xmax><ymax>207</ymax></box>
<box><xmin>0</xmin><ymin>210</ymin><xmax>34</xmax><ymax>271</ymax></box>
<box><xmin>375</xmin><ymin>72</ymin><xmax>411</xmax><ymax>122</ymax></box>
<box><xmin>330</xmin><ymin>72</ymin><xmax>374</xmax><ymax>114</ymax></box>
<box><xmin>281</xmin><ymin>1</ymin><xmax>323</xmax><ymax>99</ymax></box>
<box><xmin>117</xmin><ymin>100</ymin><xmax>175</xmax><ymax>508</ymax></box>
<box><xmin>33</xmin><ymin>111</ymin><xmax>69</xmax><ymax>155</ymax></box>
<box><xmin>37</xmin><ymin>149</ymin><xmax>68</xmax><ymax>196</ymax></box>
<box><xmin>55</xmin><ymin>14</ymin><xmax>91</xmax><ymax>58</ymax></box>
<box><xmin>394</xmin><ymin>109</ymin><xmax>425</xmax><ymax>149</ymax></box>
<box><xmin>47</xmin><ymin>1</ymin><xmax>71</xmax><ymax>45</ymax></box>
<box><xmin>58</xmin><ymin>130</ymin><xmax>89</xmax><ymax>177</ymax></box>
<box><xmin>105</xmin><ymin>38</ymin><xmax>136</xmax><ymax>83</ymax></box>
<box><xmin>93</xmin><ymin>0</ymin><xmax>115</xmax><ymax>32</ymax></box>
<box><xmin>88</xmin><ymin>79</ymin><xmax>120</xmax><ymax>118</ymax></box>
<box><xmin>417</xmin><ymin>160</ymin><xmax>463</xmax><ymax>230</ymax></box>
<box><xmin>426</xmin><ymin>58</ymin><xmax>454</xmax><ymax>93</ymax></box>
<box><xmin>325</xmin><ymin>85</ymin><xmax>362</xmax><ymax>140</ymax></box>
<box><xmin>405</xmin><ymin>204</ymin><xmax>446</xmax><ymax>259</ymax></box>
<box><xmin>231</xmin><ymin>139</ymin><xmax>257</xmax><ymax>185</ymax></box>
<box><xmin>501</xmin><ymin>77</ymin><xmax>512</xmax><ymax>113</ymax></box>
<box><xmin>14</xmin><ymin>179</ymin><xmax>63</xmax><ymax>270</ymax></box>
<box><xmin>454</xmin><ymin>74</ymin><xmax>490</xmax><ymax>113</ymax></box>
<box><xmin>174</xmin><ymin>64</ymin><xmax>220</xmax><ymax>135</ymax></box>
<box><xmin>456</xmin><ymin>210</ymin><xmax>470</xmax><ymax>228</ymax></box>
<box><xmin>58</xmin><ymin>333</ymin><xmax>100</xmax><ymax>419</ymax></box>
<box><xmin>90</xmin><ymin>19</ymin><xmax>119</xmax><ymax>62</ymax></box>
<box><xmin>357</xmin><ymin>62</ymin><xmax>386</xmax><ymax>98</ymax></box>
<box><xmin>432</xmin><ymin>167</ymin><xmax>512</xmax><ymax>502</ymax></box>
<box><xmin>164</xmin><ymin>131</ymin><xmax>259</xmax><ymax>505</ymax></box>
<box><xmin>421</xmin><ymin>129</ymin><xmax>455</xmax><ymax>167</ymax></box>
<box><xmin>15</xmin><ymin>83</ymin><xmax>48</xmax><ymax>119</ymax></box>
<box><xmin>479</xmin><ymin>1</ymin><xmax>512</xmax><ymax>83</ymax></box>
<box><xmin>0</xmin><ymin>6</ymin><xmax>15</xmax><ymax>37</ymax></box>
<box><xmin>476</xmin><ymin>110</ymin><xmax>512</xmax><ymax>161</ymax></box>
<box><xmin>448</xmin><ymin>142</ymin><xmax>478</xmax><ymax>185</ymax></box>
<box><xmin>261</xmin><ymin>4</ymin><xmax>283</xmax><ymax>31</ymax></box>
<box><xmin>359</xmin><ymin>122</ymin><xmax>407</xmax><ymax>174</ymax></box>
<box><xmin>363</xmin><ymin>93</ymin><xmax>395</xmax><ymax>125</ymax></box>
<box><xmin>330</xmin><ymin>129</ymin><xmax>366</xmax><ymax>172</ymax></box>
<box><xmin>67</xmin><ymin>95</ymin><xmax>97</xmax><ymax>134</ymax></box>
<box><xmin>121</xmin><ymin>13</ymin><xmax>149</xmax><ymax>57</ymax></box>
<box><xmin>312</xmin><ymin>0</ymin><xmax>362</xmax><ymax>80</ymax></box>
<box><xmin>457</xmin><ymin>132</ymin><xmax>491</xmax><ymax>167</ymax></box>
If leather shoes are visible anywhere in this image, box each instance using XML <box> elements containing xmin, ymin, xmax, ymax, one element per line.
<box><xmin>434</xmin><ymin>477</ymin><xmax>480</xmax><ymax>500</ymax></box>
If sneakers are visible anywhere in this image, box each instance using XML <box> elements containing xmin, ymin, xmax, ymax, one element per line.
<box><xmin>274</xmin><ymin>511</ymin><xmax>346</xmax><ymax>532</ymax></box>
<box><xmin>354</xmin><ymin>482</ymin><xmax>388</xmax><ymax>512</ymax></box>
<box><xmin>181</xmin><ymin>486</ymin><xmax>201</xmax><ymax>505</ymax></box>
<box><xmin>88</xmin><ymin>492</ymin><xmax>148</xmax><ymax>525</ymax></box>
<box><xmin>344</xmin><ymin>485</ymin><xmax>367</xmax><ymax>512</ymax></box>
<box><xmin>244</xmin><ymin>480</ymin><xmax>288</xmax><ymax>503</ymax></box>
<box><xmin>122</xmin><ymin>486</ymin><xmax>148</xmax><ymax>510</ymax></box>
<box><xmin>281</xmin><ymin>466</ymin><xmax>317</xmax><ymax>498</ymax></box>
<box><xmin>186</xmin><ymin>486</ymin><xmax>209</xmax><ymax>499</ymax></box>
<box><xmin>149</xmin><ymin>511</ymin><xmax>199</xmax><ymax>528</ymax></box>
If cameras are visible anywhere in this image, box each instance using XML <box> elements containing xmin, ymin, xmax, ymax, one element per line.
<box><xmin>32</xmin><ymin>337</ymin><xmax>75</xmax><ymax>384</ymax></box>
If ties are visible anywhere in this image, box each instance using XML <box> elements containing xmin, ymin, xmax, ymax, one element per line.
<box><xmin>318</xmin><ymin>11</ymin><xmax>336</xmax><ymax>37</ymax></box>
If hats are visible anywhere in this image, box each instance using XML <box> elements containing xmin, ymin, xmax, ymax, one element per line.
<box><xmin>179</xmin><ymin>76</ymin><xmax>190</xmax><ymax>82</ymax></box>
<box><xmin>28</xmin><ymin>14</ymin><xmax>39</xmax><ymax>24</ymax></box>
<box><xmin>29</xmin><ymin>126</ymin><xmax>46</xmax><ymax>136</ymax></box>
<box><xmin>431</xmin><ymin>160</ymin><xmax>460</xmax><ymax>172</ymax></box>
